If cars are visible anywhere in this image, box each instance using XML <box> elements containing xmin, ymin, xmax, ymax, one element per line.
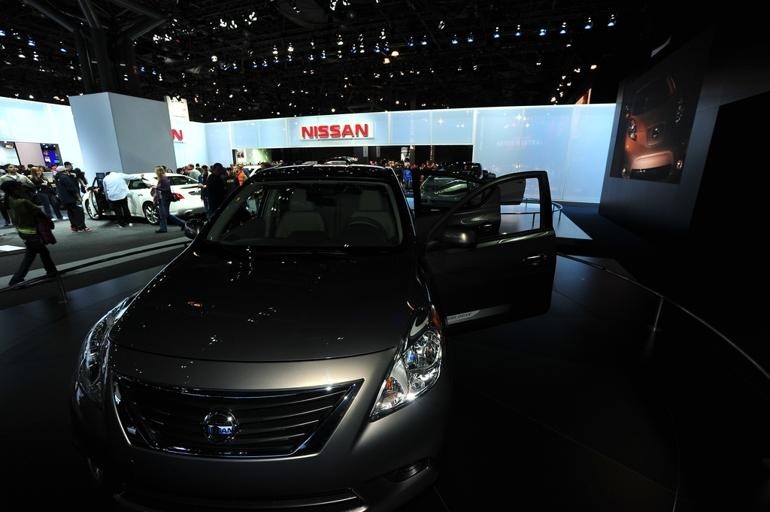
<box><xmin>81</xmin><ymin>172</ymin><xmax>206</xmax><ymax>225</ymax></box>
<box><xmin>72</xmin><ymin>164</ymin><xmax>557</xmax><ymax>512</ymax></box>
<box><xmin>621</xmin><ymin>76</ymin><xmax>690</xmax><ymax>182</ymax></box>
<box><xmin>418</xmin><ymin>164</ymin><xmax>528</xmax><ymax>238</ymax></box>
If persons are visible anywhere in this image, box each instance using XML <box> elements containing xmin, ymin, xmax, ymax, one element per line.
<box><xmin>0</xmin><ymin>162</ymin><xmax>92</xmax><ymax>233</ymax></box>
<box><xmin>152</xmin><ymin>165</ymin><xmax>173</xmax><ymax>233</ymax></box>
<box><xmin>103</xmin><ymin>171</ymin><xmax>144</xmax><ymax>228</ymax></box>
<box><xmin>176</xmin><ymin>163</ymin><xmax>249</xmax><ymax>216</ymax></box>
<box><xmin>0</xmin><ymin>181</ymin><xmax>57</xmax><ymax>287</ymax></box>
<box><xmin>368</xmin><ymin>158</ymin><xmax>446</xmax><ymax>192</ymax></box>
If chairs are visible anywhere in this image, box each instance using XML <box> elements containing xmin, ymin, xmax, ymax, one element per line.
<box><xmin>273</xmin><ymin>186</ymin><xmax>397</xmax><ymax>243</ymax></box>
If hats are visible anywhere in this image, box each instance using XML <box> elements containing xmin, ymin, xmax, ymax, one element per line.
<box><xmin>0</xmin><ymin>180</ymin><xmax>18</xmax><ymax>195</ymax></box>
